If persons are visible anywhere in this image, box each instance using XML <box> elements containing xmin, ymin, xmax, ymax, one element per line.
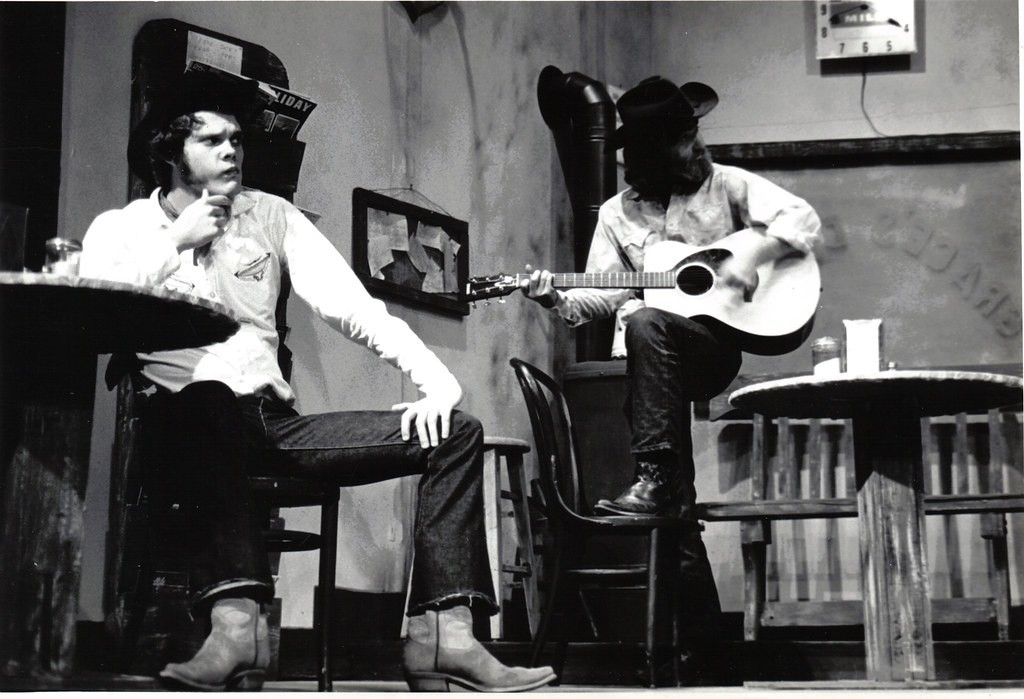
<box><xmin>521</xmin><ymin>76</ymin><xmax>823</xmax><ymax>688</ymax></box>
<box><xmin>72</xmin><ymin>70</ymin><xmax>557</xmax><ymax>692</ymax></box>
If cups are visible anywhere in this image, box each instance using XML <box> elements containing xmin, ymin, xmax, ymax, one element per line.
<box><xmin>0</xmin><ymin>205</ymin><xmax>29</xmax><ymax>271</ymax></box>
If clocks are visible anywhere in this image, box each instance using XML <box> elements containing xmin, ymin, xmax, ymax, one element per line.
<box><xmin>815</xmin><ymin>0</ymin><xmax>918</xmax><ymax>59</ymax></box>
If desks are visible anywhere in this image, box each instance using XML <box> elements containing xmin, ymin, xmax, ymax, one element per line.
<box><xmin>0</xmin><ymin>270</ymin><xmax>242</xmax><ymax>693</ymax></box>
<box><xmin>733</xmin><ymin>370</ymin><xmax>1024</xmax><ymax>692</ymax></box>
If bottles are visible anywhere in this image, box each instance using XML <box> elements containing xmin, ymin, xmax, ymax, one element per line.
<box><xmin>887</xmin><ymin>361</ymin><xmax>898</xmax><ymax>372</ymax></box>
<box><xmin>45</xmin><ymin>236</ymin><xmax>83</xmax><ymax>275</ymax></box>
<box><xmin>811</xmin><ymin>335</ymin><xmax>842</xmax><ymax>375</ymax></box>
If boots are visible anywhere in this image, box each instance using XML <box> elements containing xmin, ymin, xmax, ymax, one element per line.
<box><xmin>155</xmin><ymin>596</ymin><xmax>272</xmax><ymax>692</ymax></box>
<box><xmin>403</xmin><ymin>605</ymin><xmax>557</xmax><ymax>692</ymax></box>
<box><xmin>593</xmin><ymin>454</ymin><xmax>689</xmax><ymax>518</ymax></box>
<box><xmin>635</xmin><ymin>634</ymin><xmax>743</xmax><ymax>686</ymax></box>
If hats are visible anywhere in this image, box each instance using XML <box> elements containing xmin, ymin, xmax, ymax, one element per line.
<box><xmin>603</xmin><ymin>74</ymin><xmax>720</xmax><ymax>153</ymax></box>
<box><xmin>127</xmin><ymin>71</ymin><xmax>259</xmax><ymax>182</ymax></box>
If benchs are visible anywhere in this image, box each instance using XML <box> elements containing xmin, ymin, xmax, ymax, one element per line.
<box><xmin>696</xmin><ymin>362</ymin><xmax>1024</xmax><ymax>681</ymax></box>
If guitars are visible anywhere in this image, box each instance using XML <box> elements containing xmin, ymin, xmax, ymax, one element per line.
<box><xmin>457</xmin><ymin>225</ymin><xmax>825</xmax><ymax>357</ymax></box>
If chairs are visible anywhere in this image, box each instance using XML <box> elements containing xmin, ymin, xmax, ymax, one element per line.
<box><xmin>510</xmin><ymin>358</ymin><xmax>705</xmax><ymax>693</ymax></box>
<box><xmin>120</xmin><ymin>368</ymin><xmax>341</xmax><ymax>693</ymax></box>
